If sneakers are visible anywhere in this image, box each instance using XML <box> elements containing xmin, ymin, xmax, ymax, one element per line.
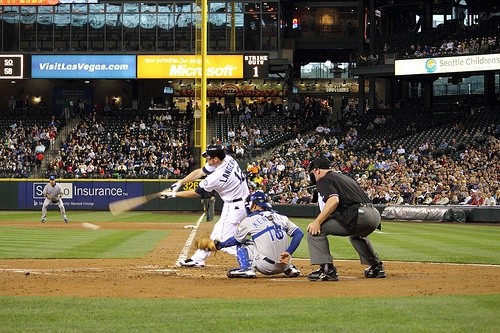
<box><xmin>308</xmin><ymin>267</ymin><xmax>338</xmax><ymax>281</ymax></box>
<box><xmin>227</xmin><ymin>268</ymin><xmax>255</xmax><ymax>277</ymax></box>
<box><xmin>364</xmin><ymin>262</ymin><xmax>386</xmax><ymax>278</ymax></box>
<box><xmin>284</xmin><ymin>264</ymin><xmax>300</xmax><ymax>277</ymax></box>
<box><xmin>179</xmin><ymin>259</ymin><xmax>204</xmax><ymax>267</ymax></box>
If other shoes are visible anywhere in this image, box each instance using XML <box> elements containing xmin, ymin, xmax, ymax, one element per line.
<box><xmin>64</xmin><ymin>218</ymin><xmax>67</xmax><ymax>223</ymax></box>
<box><xmin>41</xmin><ymin>218</ymin><xmax>46</xmax><ymax>222</ymax></box>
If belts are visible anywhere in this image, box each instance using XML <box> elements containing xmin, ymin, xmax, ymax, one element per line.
<box><xmin>233</xmin><ymin>198</ymin><xmax>242</xmax><ymax>202</ymax></box>
<box><xmin>264</xmin><ymin>257</ymin><xmax>275</xmax><ymax>265</ymax></box>
<box><xmin>359</xmin><ymin>203</ymin><xmax>373</xmax><ymax>207</ymax></box>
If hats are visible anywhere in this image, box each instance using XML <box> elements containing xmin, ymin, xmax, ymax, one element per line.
<box><xmin>202</xmin><ymin>144</ymin><xmax>222</xmax><ymax>158</ymax></box>
<box><xmin>49</xmin><ymin>176</ymin><xmax>55</xmax><ymax>180</ymax></box>
<box><xmin>310</xmin><ymin>158</ymin><xmax>332</xmax><ymax>169</ymax></box>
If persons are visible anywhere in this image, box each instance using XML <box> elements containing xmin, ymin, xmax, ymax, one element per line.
<box><xmin>307</xmin><ymin>157</ymin><xmax>387</xmax><ymax>282</ymax></box>
<box><xmin>0</xmin><ymin>35</ymin><xmax>500</xmax><ymax>207</ymax></box>
<box><xmin>197</xmin><ymin>191</ymin><xmax>305</xmax><ymax>278</ymax></box>
<box><xmin>158</xmin><ymin>143</ymin><xmax>250</xmax><ymax>267</ymax></box>
<box><xmin>41</xmin><ymin>176</ymin><xmax>68</xmax><ymax>223</ymax></box>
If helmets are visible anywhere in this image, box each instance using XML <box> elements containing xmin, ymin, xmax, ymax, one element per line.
<box><xmin>250</xmin><ymin>191</ymin><xmax>272</xmax><ymax>207</ymax></box>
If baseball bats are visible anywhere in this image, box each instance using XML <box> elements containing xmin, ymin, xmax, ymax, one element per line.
<box><xmin>108</xmin><ymin>189</ymin><xmax>172</xmax><ymax>217</ymax></box>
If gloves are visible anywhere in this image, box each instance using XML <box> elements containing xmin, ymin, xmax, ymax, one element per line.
<box><xmin>159</xmin><ymin>191</ymin><xmax>176</xmax><ymax>199</ymax></box>
<box><xmin>169</xmin><ymin>182</ymin><xmax>181</xmax><ymax>192</ymax></box>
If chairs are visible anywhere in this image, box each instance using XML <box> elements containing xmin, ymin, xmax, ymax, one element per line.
<box><xmin>0</xmin><ymin>98</ymin><xmax>500</xmax><ymax>178</ymax></box>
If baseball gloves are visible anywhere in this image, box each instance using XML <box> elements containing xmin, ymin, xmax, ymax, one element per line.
<box><xmin>52</xmin><ymin>194</ymin><xmax>61</xmax><ymax>203</ymax></box>
<box><xmin>195</xmin><ymin>237</ymin><xmax>221</xmax><ymax>253</ymax></box>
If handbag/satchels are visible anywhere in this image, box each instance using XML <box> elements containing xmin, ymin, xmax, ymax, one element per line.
<box><xmin>376</xmin><ymin>223</ymin><xmax>381</xmax><ymax>230</ymax></box>
<box><xmin>333</xmin><ymin>204</ymin><xmax>360</xmax><ymax>229</ymax></box>
